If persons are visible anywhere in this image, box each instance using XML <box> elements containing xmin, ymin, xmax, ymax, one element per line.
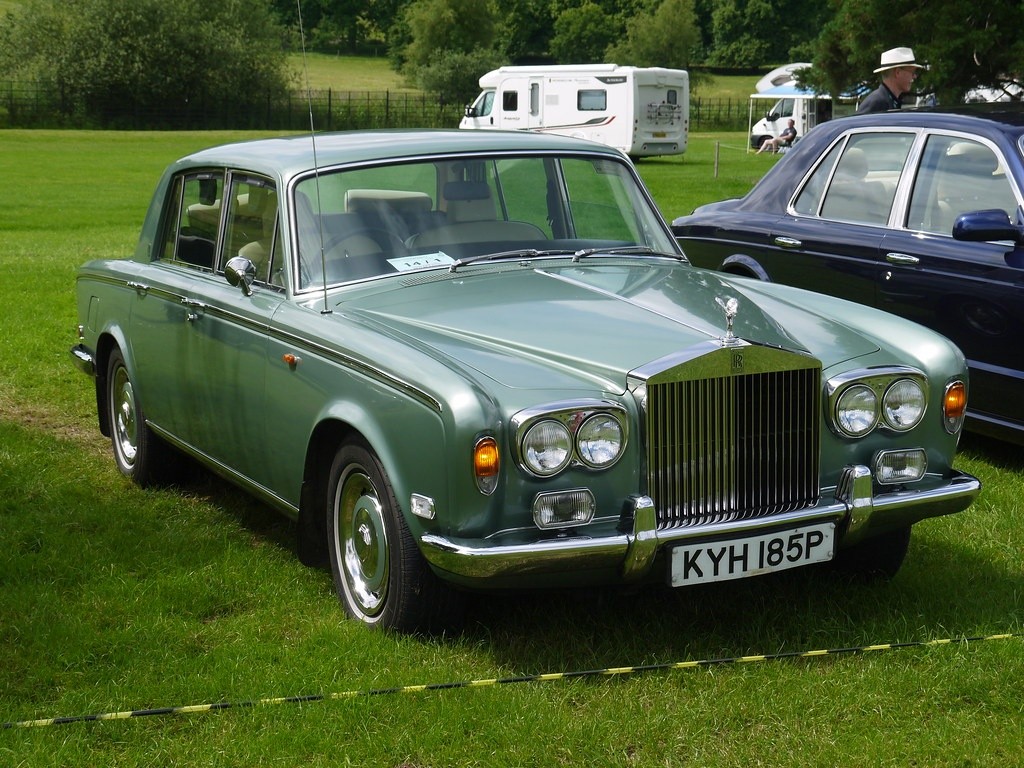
<box><xmin>756</xmin><ymin>119</ymin><xmax>797</xmax><ymax>156</ymax></box>
<box><xmin>855</xmin><ymin>46</ymin><xmax>924</xmax><ymax>115</ymax></box>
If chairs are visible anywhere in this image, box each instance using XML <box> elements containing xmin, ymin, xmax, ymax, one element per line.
<box><xmin>944</xmin><ymin>141</ymin><xmax>1004</xmax><ymax>200</ymax></box>
<box><xmin>777</xmin><ymin>136</ymin><xmax>796</xmax><ymax>154</ymax></box>
<box><xmin>236</xmin><ymin>190</ymin><xmax>383</xmax><ymax>281</ymax></box>
<box><xmin>405</xmin><ymin>195</ymin><xmax>550</xmax><ymax>250</ymax></box>
<box><xmin>819</xmin><ymin>147</ymin><xmax>891</xmax><ymax>222</ymax></box>
<box><xmin>932</xmin><ymin>156</ymin><xmax>989</xmax><ymax>233</ymax></box>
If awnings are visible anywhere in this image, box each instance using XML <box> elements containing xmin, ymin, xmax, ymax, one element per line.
<box><xmin>746</xmin><ymin>79</ymin><xmax>871</xmax><ymax>154</ymax></box>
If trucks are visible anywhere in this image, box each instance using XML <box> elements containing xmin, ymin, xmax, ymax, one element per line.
<box><xmin>457</xmin><ymin>64</ymin><xmax>691</xmax><ymax>163</ymax></box>
<box><xmin>749</xmin><ymin>63</ymin><xmax>939</xmax><ymax>150</ymax></box>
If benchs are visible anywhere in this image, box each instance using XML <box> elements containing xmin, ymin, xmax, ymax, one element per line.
<box><xmin>174</xmin><ymin>188</ymin><xmax>448</xmax><ymax>269</ymax></box>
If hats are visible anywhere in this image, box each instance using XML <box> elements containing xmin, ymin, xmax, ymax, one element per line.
<box><xmin>873</xmin><ymin>46</ymin><xmax>924</xmax><ymax>74</ymax></box>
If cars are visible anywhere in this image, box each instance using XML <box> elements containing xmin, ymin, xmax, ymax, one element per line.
<box><xmin>669</xmin><ymin>111</ymin><xmax>1024</xmax><ymax>450</ymax></box>
<box><xmin>71</xmin><ymin>128</ymin><xmax>979</xmax><ymax>633</ymax></box>
<box><xmin>965</xmin><ymin>79</ymin><xmax>1023</xmax><ymax>104</ymax></box>
